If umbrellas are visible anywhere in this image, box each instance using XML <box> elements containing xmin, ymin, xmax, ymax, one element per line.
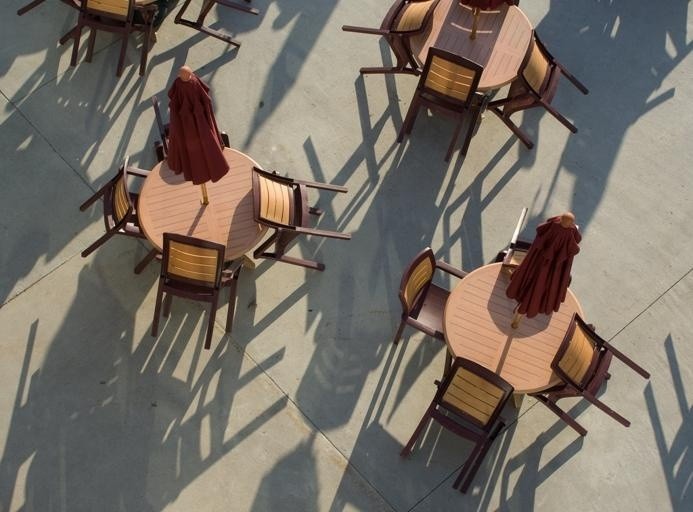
<box><xmin>461</xmin><ymin>0</ymin><xmax>500</xmax><ymax>39</ymax></box>
<box><xmin>167</xmin><ymin>66</ymin><xmax>230</xmax><ymax>205</ymax></box>
<box><xmin>506</xmin><ymin>211</ymin><xmax>582</xmax><ymax>328</ymax></box>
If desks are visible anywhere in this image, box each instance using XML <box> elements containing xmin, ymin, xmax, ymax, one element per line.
<box><xmin>407</xmin><ymin>5</ymin><xmax>534</xmax><ymax>120</ymax></box>
<box><xmin>137</xmin><ymin>147</ymin><xmax>271</xmax><ymax>269</ymax></box>
<box><xmin>444</xmin><ymin>261</ymin><xmax>585</xmax><ymax>420</ymax></box>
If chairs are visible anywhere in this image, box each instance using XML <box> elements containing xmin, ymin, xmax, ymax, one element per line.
<box><xmin>399</xmin><ymin>358</ymin><xmax>515</xmax><ymax>488</ymax></box>
<box><xmin>14</xmin><ymin>0</ymin><xmax>262</xmax><ymax>77</ymax></box>
<box><xmin>343</xmin><ymin>3</ymin><xmax>439</xmax><ymax>76</ymax></box>
<box><xmin>151</xmin><ymin>91</ymin><xmax>230</xmax><ymax>163</ymax></box>
<box><xmin>398</xmin><ymin>246</ymin><xmax>470</xmax><ymax>377</ymax></box>
<box><xmin>252</xmin><ymin>167</ymin><xmax>353</xmax><ymax>271</ymax></box>
<box><xmin>78</xmin><ymin>154</ymin><xmax>162</xmax><ymax>274</ymax></box>
<box><xmin>153</xmin><ymin>233</ymin><xmax>245</xmax><ymax>347</ymax></box>
<box><xmin>397</xmin><ymin>47</ymin><xmax>489</xmax><ymax>160</ymax></box>
<box><xmin>528</xmin><ymin>312</ymin><xmax>650</xmax><ymax>436</ymax></box>
<box><xmin>486</xmin><ymin>29</ymin><xmax>589</xmax><ymax>149</ymax></box>
<box><xmin>498</xmin><ymin>242</ymin><xmax>531</xmax><ymax>269</ymax></box>
